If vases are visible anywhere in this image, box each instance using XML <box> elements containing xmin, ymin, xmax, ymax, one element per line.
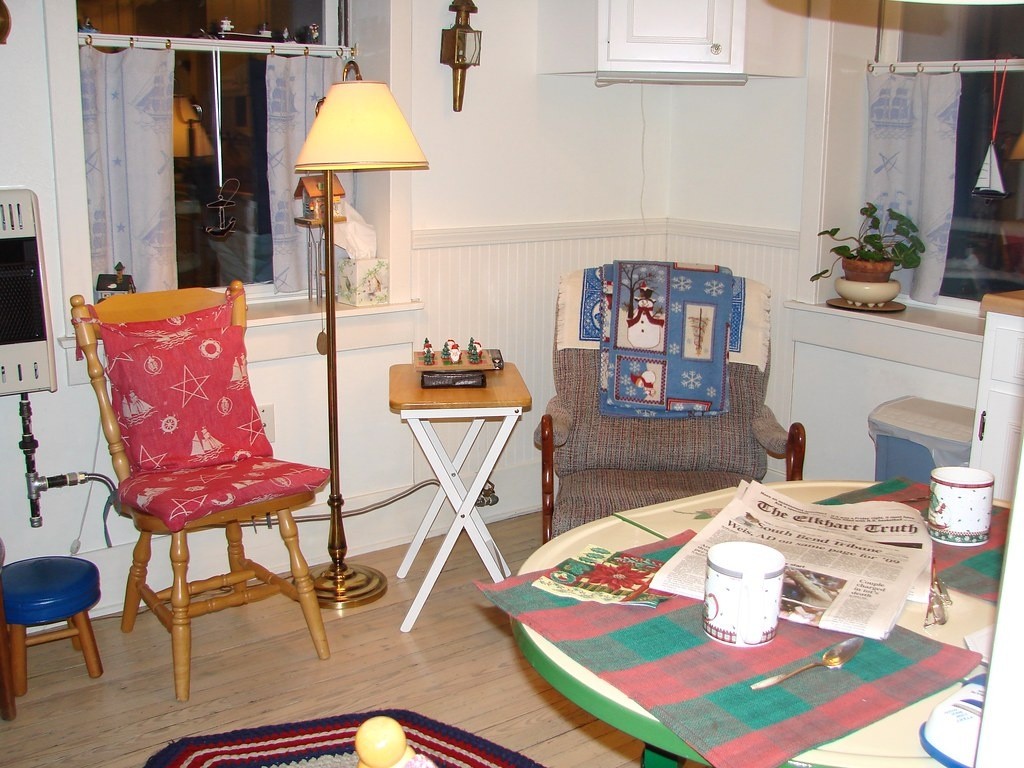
<box><xmin>810</xmin><ymin>202</ymin><xmax>922</xmax><ymax>304</ymax></box>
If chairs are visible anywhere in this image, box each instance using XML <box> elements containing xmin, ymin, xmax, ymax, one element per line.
<box><xmin>534</xmin><ymin>261</ymin><xmax>803</xmax><ymax>537</ymax></box>
<box><xmin>70</xmin><ymin>280</ymin><xmax>332</xmax><ymax>701</ymax></box>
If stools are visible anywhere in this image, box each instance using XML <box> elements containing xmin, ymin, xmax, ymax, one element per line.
<box><xmin>2</xmin><ymin>556</ymin><xmax>104</xmax><ymax>697</ymax></box>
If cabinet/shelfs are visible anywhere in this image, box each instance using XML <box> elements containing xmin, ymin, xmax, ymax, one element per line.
<box><xmin>595</xmin><ymin>1</ymin><xmax>747</xmax><ymax>89</ymax></box>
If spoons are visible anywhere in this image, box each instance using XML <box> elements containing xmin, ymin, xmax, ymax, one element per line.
<box><xmin>749</xmin><ymin>637</ymin><xmax>864</xmax><ymax>690</ymax></box>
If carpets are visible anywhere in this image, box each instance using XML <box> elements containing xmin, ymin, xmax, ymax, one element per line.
<box><xmin>145</xmin><ymin>708</ymin><xmax>544</xmax><ymax>768</ymax></box>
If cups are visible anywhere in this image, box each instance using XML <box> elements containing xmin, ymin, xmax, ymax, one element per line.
<box><xmin>702</xmin><ymin>541</ymin><xmax>785</xmax><ymax>647</ymax></box>
<box><xmin>928</xmin><ymin>465</ymin><xmax>993</xmax><ymax>546</ymax></box>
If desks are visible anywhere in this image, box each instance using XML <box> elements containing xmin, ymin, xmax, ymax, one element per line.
<box><xmin>508</xmin><ymin>479</ymin><xmax>1024</xmax><ymax>768</ymax></box>
<box><xmin>389</xmin><ymin>365</ymin><xmax>533</xmax><ymax>632</ymax></box>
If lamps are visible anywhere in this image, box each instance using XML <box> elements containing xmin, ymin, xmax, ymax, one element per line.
<box><xmin>294</xmin><ymin>62</ymin><xmax>429</xmax><ymax>611</ymax></box>
<box><xmin>441</xmin><ymin>4</ymin><xmax>483</xmax><ymax>111</ymax></box>
